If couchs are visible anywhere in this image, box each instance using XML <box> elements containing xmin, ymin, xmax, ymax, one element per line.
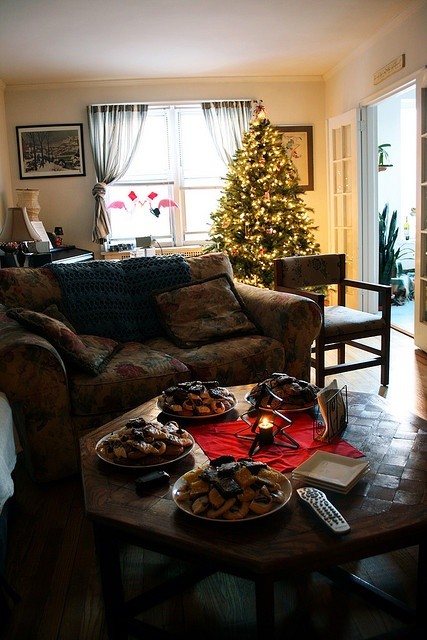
<box><xmin>0</xmin><ymin>251</ymin><xmax>323</xmax><ymax>485</ymax></box>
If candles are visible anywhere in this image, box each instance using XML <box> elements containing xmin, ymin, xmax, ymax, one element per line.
<box><xmin>256</xmin><ymin>416</ymin><xmax>275</xmax><ymax>446</ymax></box>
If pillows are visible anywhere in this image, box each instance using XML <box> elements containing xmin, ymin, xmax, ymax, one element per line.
<box><xmin>146</xmin><ymin>272</ymin><xmax>262</xmax><ymax>350</ymax></box>
<box><xmin>3</xmin><ymin>301</ymin><xmax>124</xmax><ymax>377</ymax></box>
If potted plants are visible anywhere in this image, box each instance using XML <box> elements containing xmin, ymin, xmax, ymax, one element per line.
<box><xmin>377</xmin><ymin>202</ymin><xmax>415</xmax><ymax>310</ymax></box>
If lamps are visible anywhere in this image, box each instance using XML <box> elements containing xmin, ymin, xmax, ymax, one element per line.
<box><xmin>0</xmin><ymin>205</ymin><xmax>41</xmax><ymax>268</ymax></box>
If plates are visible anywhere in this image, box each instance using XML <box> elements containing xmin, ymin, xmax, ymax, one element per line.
<box><xmin>291</xmin><ymin>465</ymin><xmax>371</xmax><ymax>492</ymax></box>
<box><xmin>156</xmin><ymin>396</ymin><xmax>239</xmax><ymax>419</ymax></box>
<box><xmin>291</xmin><ymin>449</ymin><xmax>370</xmax><ymax>488</ymax></box>
<box><xmin>292</xmin><ymin>468</ymin><xmax>371</xmax><ymax>496</ymax></box>
<box><xmin>171</xmin><ymin>465</ymin><xmax>294</xmax><ymax>524</ymax></box>
<box><xmin>244</xmin><ymin>389</ymin><xmax>318</xmax><ymax>414</ymax></box>
<box><xmin>94</xmin><ymin>423</ymin><xmax>196</xmax><ymax>469</ymax></box>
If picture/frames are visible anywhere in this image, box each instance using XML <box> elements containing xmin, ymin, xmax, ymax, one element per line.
<box><xmin>269</xmin><ymin>125</ymin><xmax>314</xmax><ymax>194</ymax></box>
<box><xmin>15</xmin><ymin>122</ymin><xmax>87</xmax><ymax>181</ymax></box>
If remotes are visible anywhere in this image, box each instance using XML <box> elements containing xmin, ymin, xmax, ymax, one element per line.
<box><xmin>297</xmin><ymin>487</ymin><xmax>351</xmax><ymax>536</ymax></box>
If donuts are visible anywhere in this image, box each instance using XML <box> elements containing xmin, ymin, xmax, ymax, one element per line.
<box><xmin>178</xmin><ymin>455</ymin><xmax>285</xmax><ymax>519</ymax></box>
<box><xmin>248</xmin><ymin>372</ymin><xmax>317</xmax><ymax>409</ymax></box>
<box><xmin>158</xmin><ymin>382</ymin><xmax>235</xmax><ymax>417</ymax></box>
<box><xmin>97</xmin><ymin>419</ymin><xmax>193</xmax><ymax>465</ymax></box>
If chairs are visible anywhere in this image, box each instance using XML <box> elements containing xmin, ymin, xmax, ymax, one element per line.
<box><xmin>271</xmin><ymin>252</ymin><xmax>392</xmax><ymax>389</ymax></box>
